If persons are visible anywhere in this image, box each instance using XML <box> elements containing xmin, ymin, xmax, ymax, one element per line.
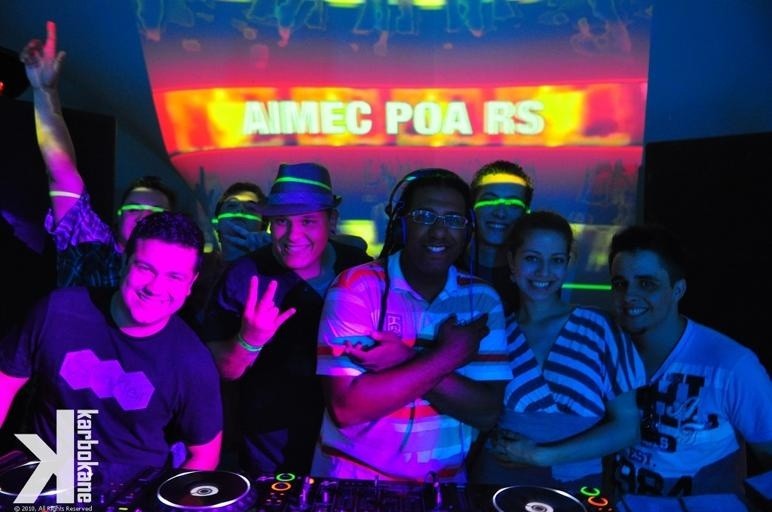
<box><xmin>1</xmin><ymin>21</ymin><xmax>772</xmax><ymax>477</ymax></box>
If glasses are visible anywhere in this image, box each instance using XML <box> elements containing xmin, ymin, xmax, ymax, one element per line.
<box><xmin>400</xmin><ymin>207</ymin><xmax>470</xmax><ymax>232</ymax></box>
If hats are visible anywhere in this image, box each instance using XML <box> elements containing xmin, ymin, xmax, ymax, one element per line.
<box><xmin>247</xmin><ymin>160</ymin><xmax>343</xmax><ymax>223</ymax></box>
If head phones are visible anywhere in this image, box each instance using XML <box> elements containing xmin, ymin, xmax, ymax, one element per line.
<box><xmin>385</xmin><ymin>168</ymin><xmax>477</xmax><ymax>249</ymax></box>
